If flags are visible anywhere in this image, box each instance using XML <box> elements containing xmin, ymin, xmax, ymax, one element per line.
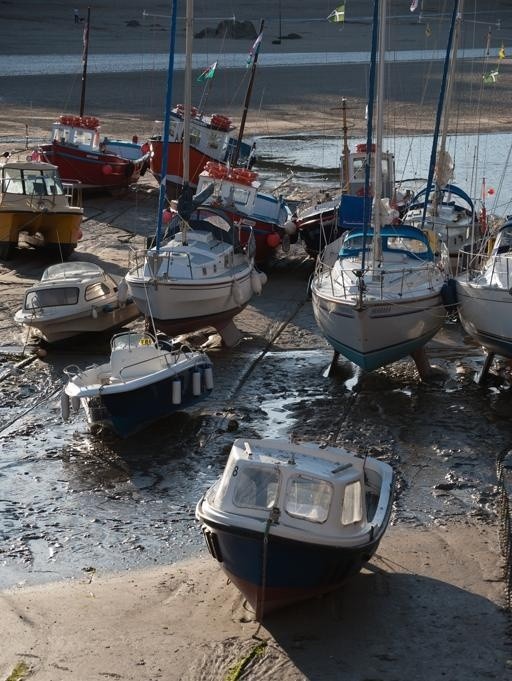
<box><xmin>424</xmin><ymin>22</ymin><xmax>434</xmax><ymax>37</ymax></box>
<box><xmin>410</xmin><ymin>0</ymin><xmax>418</xmax><ymax>11</ymax></box>
<box><xmin>418</xmin><ymin>11</ymin><xmax>425</xmax><ymax>24</ymax></box>
<box><xmin>196</xmin><ymin>59</ymin><xmax>223</xmax><ymax>80</ymax></box>
<box><xmin>499</xmin><ymin>41</ymin><xmax>507</xmax><ymax>60</ymax></box>
<box><xmin>246</xmin><ymin>31</ymin><xmax>265</xmax><ymax>70</ymax></box>
<box><xmin>328</xmin><ymin>3</ymin><xmax>344</xmax><ymax>23</ymax></box>
<box><xmin>482</xmin><ymin>67</ymin><xmax>501</xmax><ymax>83</ymax></box>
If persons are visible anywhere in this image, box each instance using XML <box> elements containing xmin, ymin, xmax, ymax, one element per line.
<box><xmin>72</xmin><ymin>7</ymin><xmax>79</xmax><ymax>23</ymax></box>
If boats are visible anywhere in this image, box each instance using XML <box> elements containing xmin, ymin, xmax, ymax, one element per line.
<box><xmin>194</xmin><ymin>438</ymin><xmax>396</xmax><ymax>623</ymax></box>
<box><xmin>63</xmin><ymin>325</ymin><xmax>223</xmax><ymax>427</ymax></box>
